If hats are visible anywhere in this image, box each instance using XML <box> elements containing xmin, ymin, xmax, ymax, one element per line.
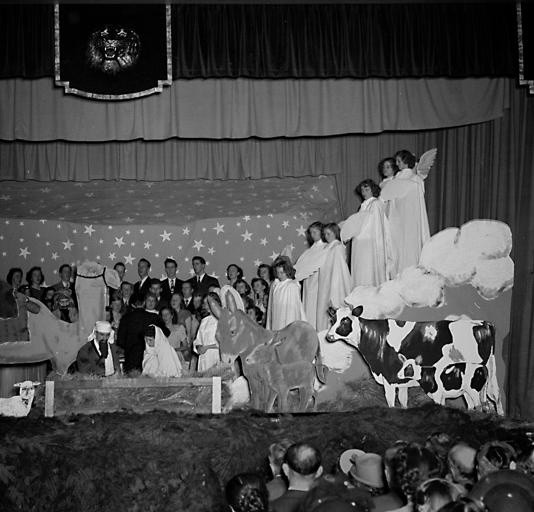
<box><xmin>471</xmin><ymin>470</ymin><xmax>534</xmax><ymax>512</ymax></box>
<box><xmin>350</xmin><ymin>453</ymin><xmax>386</xmax><ymax>488</ymax></box>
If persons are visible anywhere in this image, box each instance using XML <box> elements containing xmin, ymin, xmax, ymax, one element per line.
<box><xmin>220</xmin><ymin>421</ymin><xmax>534</xmax><ymax>512</ymax></box>
<box><xmin>295</xmin><ymin>221</ymin><xmax>353</xmax><ymax>334</ymax></box>
<box><xmin>377</xmin><ymin>149</ymin><xmax>430</xmax><ymax>278</ymax></box>
<box><xmin>65</xmin><ymin>256</ymin><xmax>306</xmax><ymax>377</ymax></box>
<box><xmin>376</xmin><ymin>157</ymin><xmax>397</xmax><ymax>185</ymax></box>
<box><xmin>336</xmin><ymin>176</ymin><xmax>386</xmax><ymax>289</ymax></box>
<box><xmin>2</xmin><ymin>263</ymin><xmax>78</xmax><ymax>326</ymax></box>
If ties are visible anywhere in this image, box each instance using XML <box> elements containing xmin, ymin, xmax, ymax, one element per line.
<box><xmin>186</xmin><ymin>300</ymin><xmax>188</xmax><ymax>308</ymax></box>
<box><xmin>171</xmin><ymin>279</ymin><xmax>174</xmax><ymax>294</ymax></box>
<box><xmin>198</xmin><ymin>276</ymin><xmax>200</xmax><ymax>284</ymax></box>
